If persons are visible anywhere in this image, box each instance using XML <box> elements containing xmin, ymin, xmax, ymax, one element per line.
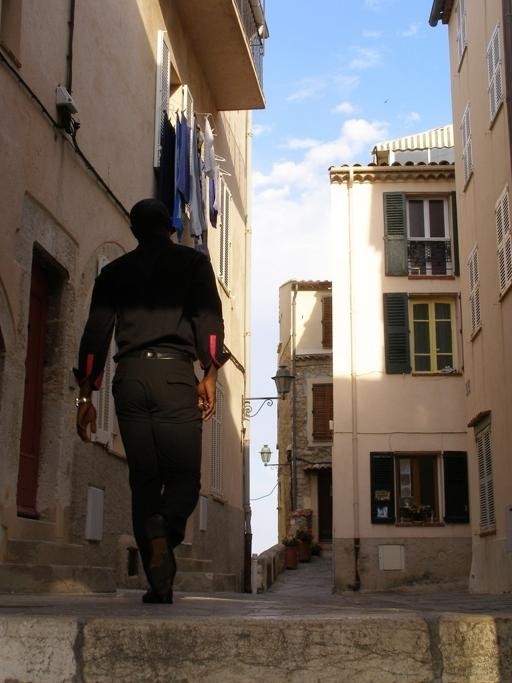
<box><xmin>72</xmin><ymin>197</ymin><xmax>233</xmax><ymax>605</ymax></box>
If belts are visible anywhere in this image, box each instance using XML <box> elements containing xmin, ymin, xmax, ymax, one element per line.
<box><xmin>122</xmin><ymin>351</ymin><xmax>192</xmax><ymax>363</ymax></box>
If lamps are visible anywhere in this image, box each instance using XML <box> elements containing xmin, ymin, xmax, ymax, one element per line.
<box><xmin>241</xmin><ymin>364</ymin><xmax>296</xmax><ymax>429</ymax></box>
<box><xmin>258</xmin><ymin>445</ymin><xmax>292</xmax><ymax>467</ymax></box>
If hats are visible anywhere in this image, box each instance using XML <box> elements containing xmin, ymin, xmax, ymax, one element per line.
<box><xmin>130</xmin><ymin>199</ymin><xmax>175</xmax><ymax>235</ymax></box>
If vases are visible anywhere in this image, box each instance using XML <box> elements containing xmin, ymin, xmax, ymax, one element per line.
<box><xmin>286</xmin><ymin>544</ymin><xmax>311</xmax><ymax>569</ymax></box>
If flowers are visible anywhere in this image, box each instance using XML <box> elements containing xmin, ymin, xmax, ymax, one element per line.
<box><xmin>283</xmin><ymin>506</ymin><xmax>314</xmax><ymax>547</ymax></box>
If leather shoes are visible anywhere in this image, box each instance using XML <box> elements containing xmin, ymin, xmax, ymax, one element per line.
<box><xmin>141</xmin><ymin>515</ymin><xmax>177</xmax><ymax>603</ymax></box>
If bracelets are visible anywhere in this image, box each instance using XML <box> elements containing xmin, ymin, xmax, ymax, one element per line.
<box><xmin>75</xmin><ymin>393</ymin><xmax>92</xmax><ymax>408</ymax></box>
